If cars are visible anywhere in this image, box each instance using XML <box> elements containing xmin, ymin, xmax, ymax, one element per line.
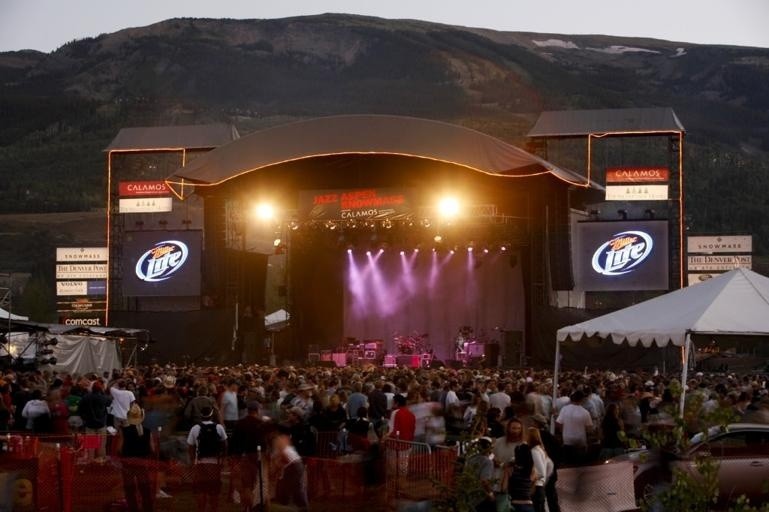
<box><xmin>599</xmin><ymin>422</ymin><xmax>767</xmax><ymax>511</ymax></box>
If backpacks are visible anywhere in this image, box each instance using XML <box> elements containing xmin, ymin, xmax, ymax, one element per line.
<box><xmin>197</xmin><ymin>422</ymin><xmax>221</xmax><ymax>460</ymax></box>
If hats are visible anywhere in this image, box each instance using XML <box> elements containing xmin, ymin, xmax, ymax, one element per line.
<box><xmin>286</xmin><ymin>406</ymin><xmax>304</xmax><ymax>417</ymax></box>
<box><xmin>163</xmin><ymin>375</ymin><xmax>176</xmax><ymax>388</ymax></box>
<box><xmin>528</xmin><ymin>414</ymin><xmax>547</xmax><ymax>424</ymax></box>
<box><xmin>198</xmin><ymin>386</ymin><xmax>208</xmax><ymax>395</ymax></box>
<box><xmin>127</xmin><ymin>403</ymin><xmax>144</xmax><ymax>425</ymax></box>
<box><xmin>247</xmin><ymin>400</ymin><xmax>262</xmax><ymax>410</ymax></box>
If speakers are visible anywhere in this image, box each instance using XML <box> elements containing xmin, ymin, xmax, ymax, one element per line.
<box><xmin>548</xmin><ymin>184</ymin><xmax>576</xmax><ymax>291</ymax></box>
<box><xmin>316</xmin><ymin>360</ymin><xmax>336</xmax><ymax>368</ymax></box>
<box><xmin>422</xmin><ymin>359</ymin><xmax>445</xmax><ymax>369</ymax></box>
<box><xmin>446</xmin><ymin>359</ymin><xmax>463</xmax><ymax>369</ymax></box>
<box><xmin>359</xmin><ymin>359</ymin><xmax>378</xmax><ymax>368</ymax></box>
<box><xmin>501</xmin><ymin>330</ymin><xmax>522</xmax><ymax>369</ymax></box>
<box><xmin>204</xmin><ymin>192</ymin><xmax>226</xmax><ymax>296</ymax></box>
